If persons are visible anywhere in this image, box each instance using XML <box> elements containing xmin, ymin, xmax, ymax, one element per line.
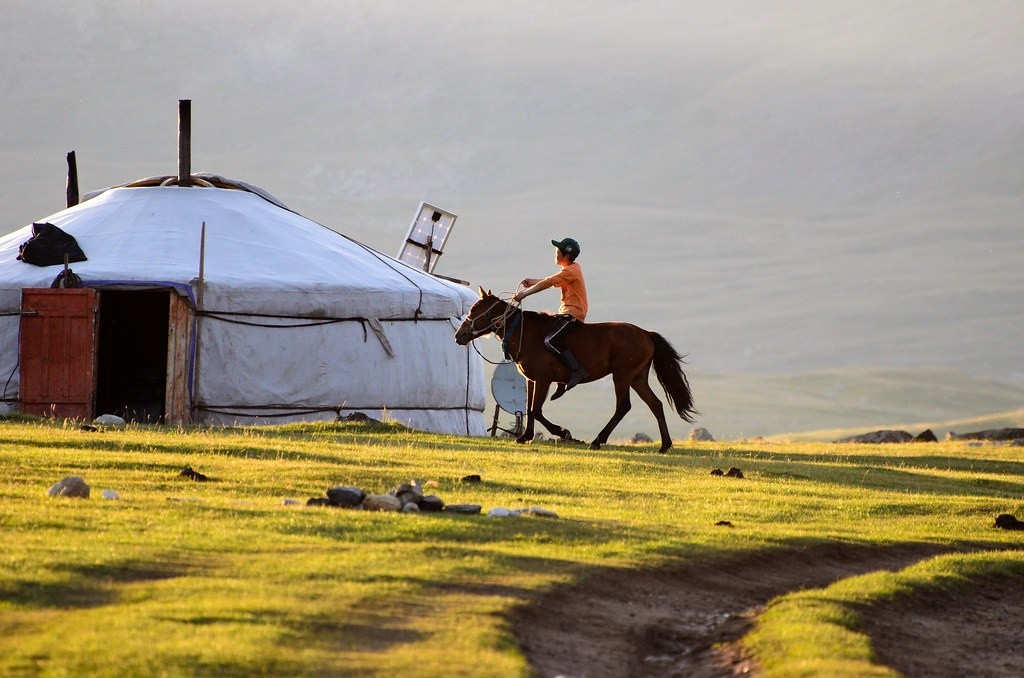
<box><xmin>514</xmin><ymin>238</ymin><xmax>588</xmax><ymax>401</ymax></box>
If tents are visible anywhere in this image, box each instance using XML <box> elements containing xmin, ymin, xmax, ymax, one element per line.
<box><xmin>0</xmin><ymin>169</ymin><xmax>488</xmax><ymax>438</ymax></box>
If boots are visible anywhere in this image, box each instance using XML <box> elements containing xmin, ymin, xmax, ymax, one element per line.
<box><xmin>552</xmin><ymin>383</ymin><xmax>567</xmax><ymax>400</ymax></box>
<box><xmin>555</xmin><ymin>348</ymin><xmax>589</xmax><ymax>390</ymax></box>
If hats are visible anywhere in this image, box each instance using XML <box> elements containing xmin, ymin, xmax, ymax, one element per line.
<box><xmin>551</xmin><ymin>238</ymin><xmax>580</xmax><ymax>258</ymax></box>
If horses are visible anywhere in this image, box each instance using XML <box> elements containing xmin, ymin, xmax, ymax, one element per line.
<box><xmin>454</xmin><ymin>285</ymin><xmax>705</xmax><ymax>454</ymax></box>
